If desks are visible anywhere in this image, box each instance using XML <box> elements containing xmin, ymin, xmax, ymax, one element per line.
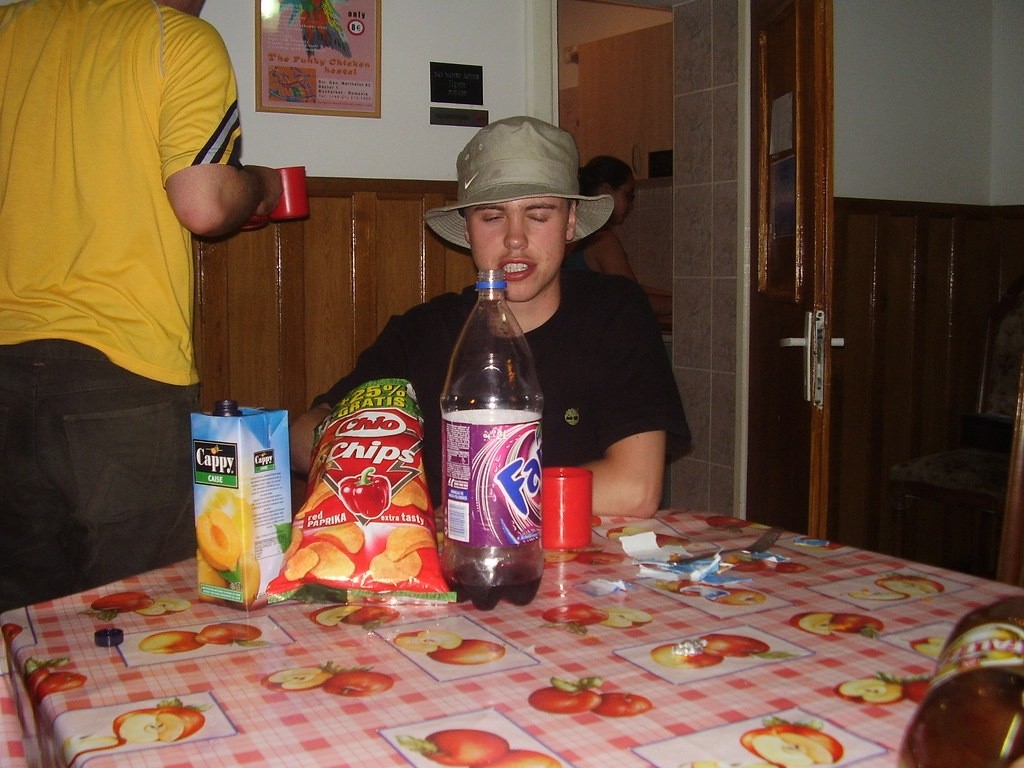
<box><xmin>0</xmin><ymin>501</ymin><xmax>1024</xmax><ymax>768</ymax></box>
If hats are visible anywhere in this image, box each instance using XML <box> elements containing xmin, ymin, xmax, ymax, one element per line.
<box><xmin>424</xmin><ymin>115</ymin><xmax>615</xmax><ymax>248</ymax></box>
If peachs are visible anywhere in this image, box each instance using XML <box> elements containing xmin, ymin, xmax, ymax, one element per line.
<box><xmin>195</xmin><ymin>492</ymin><xmax>260</xmax><ymax>608</ymax></box>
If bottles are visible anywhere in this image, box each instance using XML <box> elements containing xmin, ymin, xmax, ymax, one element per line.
<box><xmin>439</xmin><ymin>267</ymin><xmax>545</xmax><ymax>611</ymax></box>
<box><xmin>895</xmin><ymin>595</ymin><xmax>1024</xmax><ymax>768</ymax></box>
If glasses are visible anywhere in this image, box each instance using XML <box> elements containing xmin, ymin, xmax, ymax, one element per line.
<box><xmin>615</xmin><ymin>186</ymin><xmax>635</xmax><ymax>202</ymax></box>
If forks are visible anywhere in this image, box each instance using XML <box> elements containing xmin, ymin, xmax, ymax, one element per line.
<box><xmin>668</xmin><ymin>527</ymin><xmax>785</xmax><ymax>565</ymax></box>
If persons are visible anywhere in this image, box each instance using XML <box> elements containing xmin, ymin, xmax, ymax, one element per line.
<box><xmin>288</xmin><ymin>116</ymin><xmax>691</xmax><ymax>517</ymax></box>
<box><xmin>0</xmin><ymin>0</ymin><xmax>284</xmax><ymax>610</ymax></box>
<box><xmin>567</xmin><ymin>154</ymin><xmax>673</xmax><ymax>319</ymax></box>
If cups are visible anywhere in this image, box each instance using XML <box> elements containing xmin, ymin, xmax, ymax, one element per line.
<box><xmin>542</xmin><ymin>466</ymin><xmax>592</xmax><ymax>550</ymax></box>
<box><xmin>240</xmin><ymin>211</ymin><xmax>269</xmax><ymax>230</ymax></box>
<box><xmin>270</xmin><ymin>165</ymin><xmax>310</xmax><ymax>221</ymax></box>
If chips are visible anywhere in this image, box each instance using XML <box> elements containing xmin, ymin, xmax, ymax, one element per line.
<box><xmin>283</xmin><ymin>455</ymin><xmax>436</xmax><ymax>585</ymax></box>
